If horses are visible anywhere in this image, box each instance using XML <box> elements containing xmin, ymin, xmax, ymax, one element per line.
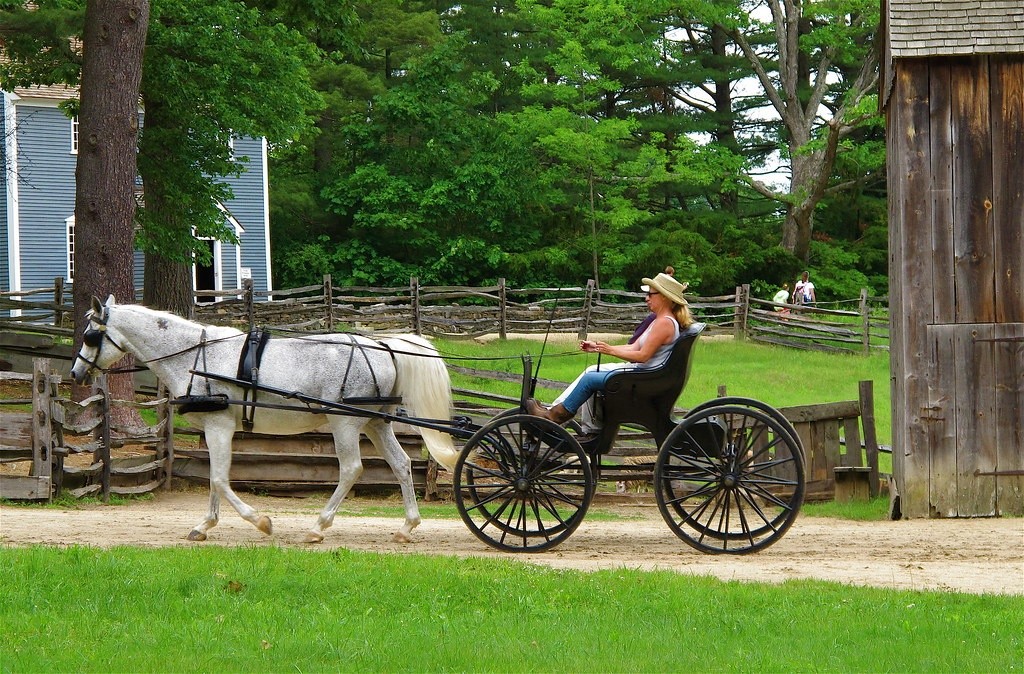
<box><xmin>71</xmin><ymin>293</ymin><xmax>478</xmax><ymax>543</ymax></box>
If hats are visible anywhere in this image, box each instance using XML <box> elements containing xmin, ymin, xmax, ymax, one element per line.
<box><xmin>642</xmin><ymin>273</ymin><xmax>688</xmax><ymax>306</ymax></box>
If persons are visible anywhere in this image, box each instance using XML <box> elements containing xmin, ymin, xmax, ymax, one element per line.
<box><xmin>773</xmin><ymin>283</ymin><xmax>791</xmax><ymax>324</ymax></box>
<box><xmin>539</xmin><ymin>283</ymin><xmax>694</xmax><ymax>443</ymax></box>
<box><xmin>524</xmin><ymin>272</ymin><xmax>691</xmax><ymax>434</ymax></box>
<box><xmin>792</xmin><ymin>270</ymin><xmax>818</xmax><ymax>316</ymax></box>
<box><xmin>665</xmin><ymin>265</ymin><xmax>689</xmax><ymax>292</ymax></box>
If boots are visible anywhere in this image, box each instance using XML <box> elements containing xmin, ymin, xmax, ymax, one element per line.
<box><xmin>526</xmin><ymin>398</ymin><xmax>574</xmax><ymax>425</ymax></box>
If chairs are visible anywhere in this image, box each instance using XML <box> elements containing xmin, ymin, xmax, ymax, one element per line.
<box><xmin>594</xmin><ymin>322</ymin><xmax>708</xmax><ymax>427</ymax></box>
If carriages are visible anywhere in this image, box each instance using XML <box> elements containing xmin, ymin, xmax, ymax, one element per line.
<box><xmin>73</xmin><ymin>286</ymin><xmax>806</xmax><ymax>557</ymax></box>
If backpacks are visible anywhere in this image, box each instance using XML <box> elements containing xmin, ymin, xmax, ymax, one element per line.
<box><xmin>773</xmin><ymin>295</ymin><xmax>786</xmax><ymax>311</ymax></box>
<box><xmin>792</xmin><ymin>283</ymin><xmax>808</xmax><ymax>304</ymax></box>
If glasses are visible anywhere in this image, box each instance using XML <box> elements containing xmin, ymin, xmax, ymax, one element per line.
<box><xmin>649</xmin><ymin>291</ymin><xmax>661</xmax><ymax>298</ymax></box>
